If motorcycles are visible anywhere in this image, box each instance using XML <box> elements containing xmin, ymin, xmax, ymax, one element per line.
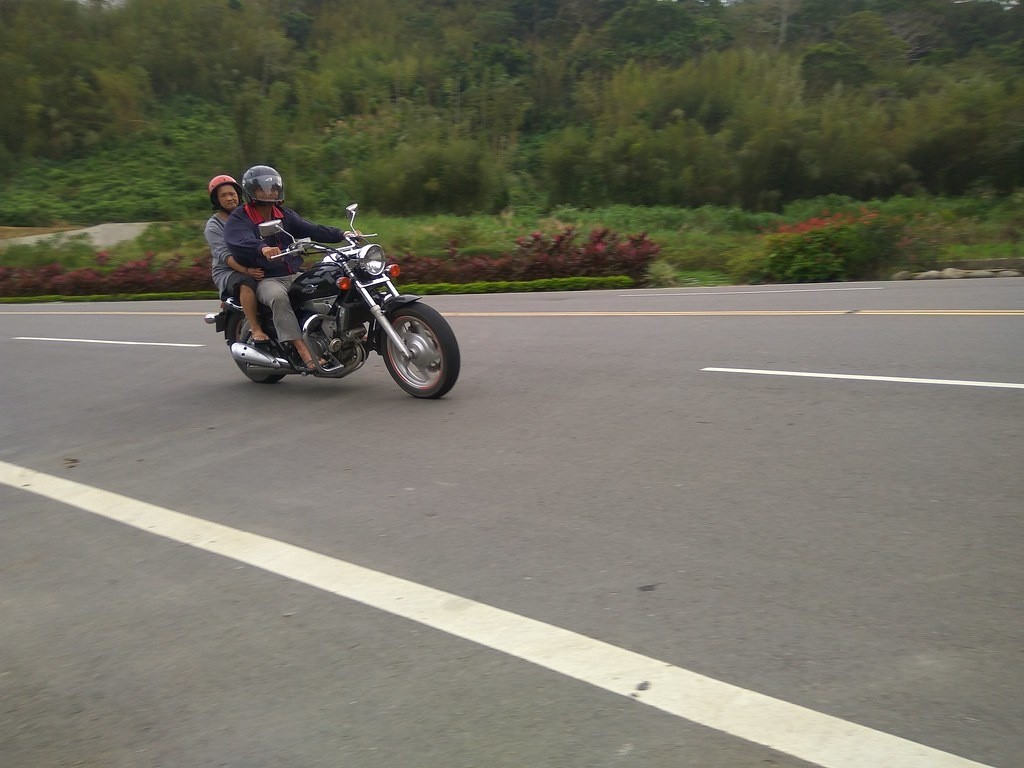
<box><xmin>204</xmin><ymin>203</ymin><xmax>462</xmax><ymax>400</ymax></box>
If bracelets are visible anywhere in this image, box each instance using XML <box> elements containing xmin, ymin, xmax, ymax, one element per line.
<box><xmin>246</xmin><ymin>267</ymin><xmax>248</xmax><ymax>275</ymax></box>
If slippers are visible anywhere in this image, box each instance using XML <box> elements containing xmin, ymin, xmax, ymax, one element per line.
<box><xmin>251</xmin><ymin>334</ymin><xmax>270</xmax><ymax>343</ymax></box>
<box><xmin>303</xmin><ymin>357</ymin><xmax>326</xmax><ymax>370</ymax></box>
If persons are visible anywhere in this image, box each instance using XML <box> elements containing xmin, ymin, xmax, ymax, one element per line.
<box><xmin>223</xmin><ymin>165</ymin><xmax>363</xmax><ymax>370</ymax></box>
<box><xmin>204</xmin><ymin>175</ymin><xmax>271</xmax><ymax>343</ymax></box>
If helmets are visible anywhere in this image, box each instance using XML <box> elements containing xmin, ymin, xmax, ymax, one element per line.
<box><xmin>208</xmin><ymin>175</ymin><xmax>243</xmax><ymax>208</ymax></box>
<box><xmin>243</xmin><ymin>165</ymin><xmax>284</xmax><ymax>205</ymax></box>
